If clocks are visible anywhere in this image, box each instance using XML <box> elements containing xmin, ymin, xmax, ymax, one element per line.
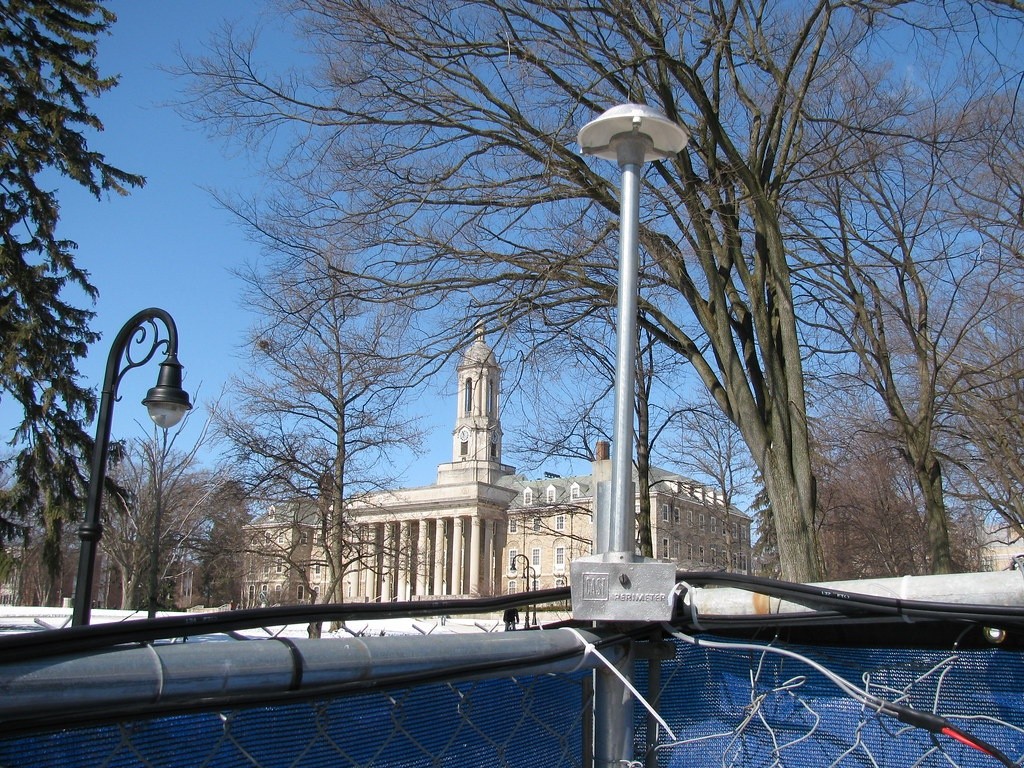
<box><xmin>460</xmin><ymin>430</ymin><xmax>469</xmax><ymax>441</ymax></box>
<box><xmin>492</xmin><ymin>432</ymin><xmax>498</xmax><ymax>442</ymax></box>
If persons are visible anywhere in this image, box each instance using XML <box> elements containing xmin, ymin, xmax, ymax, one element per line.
<box><xmin>503</xmin><ymin>608</ymin><xmax>519</xmax><ymax>631</ymax></box>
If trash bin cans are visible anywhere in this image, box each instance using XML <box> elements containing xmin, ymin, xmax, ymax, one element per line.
<box><xmin>63</xmin><ymin>597</ymin><xmax>74</xmax><ymax>608</ymax></box>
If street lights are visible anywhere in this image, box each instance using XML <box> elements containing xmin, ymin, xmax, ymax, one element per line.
<box><xmin>70</xmin><ymin>306</ymin><xmax>192</xmax><ymax>626</ymax></box>
<box><xmin>506</xmin><ymin>553</ymin><xmax>537</xmax><ymax>631</ymax></box>
